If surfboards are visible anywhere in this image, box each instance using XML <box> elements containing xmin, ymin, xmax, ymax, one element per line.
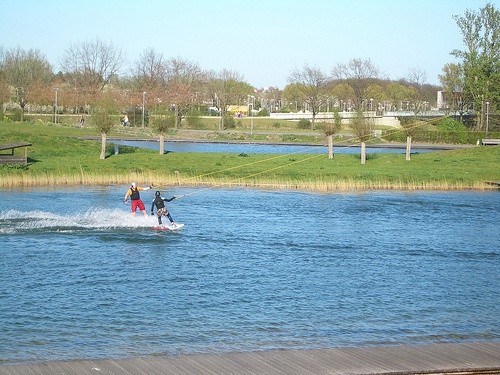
<box><xmin>151</xmin><ymin>223</ymin><xmax>184</xmax><ymax>231</ymax></box>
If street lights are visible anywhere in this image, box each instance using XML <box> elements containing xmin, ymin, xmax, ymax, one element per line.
<box><xmin>251</xmin><ymin>95</ymin><xmax>255</xmax><ymax>135</ymax></box>
<box><xmin>142</xmin><ymin>91</ymin><xmax>147</xmax><ymax>129</ymax></box>
<box><xmin>369</xmin><ymin>98</ymin><xmax>374</xmax><ymax>124</ymax></box>
<box><xmin>485</xmin><ymin>101</ymin><xmax>490</xmax><ymax>132</ymax></box>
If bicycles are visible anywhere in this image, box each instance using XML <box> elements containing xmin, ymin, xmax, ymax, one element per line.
<box><xmin>122</xmin><ymin>121</ymin><xmax>131</xmax><ymax>127</ymax></box>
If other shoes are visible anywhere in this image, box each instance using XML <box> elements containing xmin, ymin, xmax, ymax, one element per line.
<box><xmin>171</xmin><ymin>222</ymin><xmax>177</xmax><ymax>228</ymax></box>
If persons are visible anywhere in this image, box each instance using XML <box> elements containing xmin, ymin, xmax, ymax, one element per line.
<box><xmin>151</xmin><ymin>191</ymin><xmax>177</xmax><ymax>228</ymax></box>
<box><xmin>235</xmin><ymin>111</ymin><xmax>242</xmax><ymax>118</ymax></box>
<box><xmin>80</xmin><ymin>116</ymin><xmax>85</xmax><ymax>125</ymax></box>
<box><xmin>122</xmin><ymin>114</ymin><xmax>128</xmax><ymax>126</ymax></box>
<box><xmin>124</xmin><ymin>183</ymin><xmax>153</xmax><ymax>216</ymax></box>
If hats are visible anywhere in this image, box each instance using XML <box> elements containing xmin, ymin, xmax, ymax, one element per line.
<box><xmin>155</xmin><ymin>191</ymin><xmax>161</xmax><ymax>196</ymax></box>
<box><xmin>131</xmin><ymin>183</ymin><xmax>136</xmax><ymax>186</ymax></box>
